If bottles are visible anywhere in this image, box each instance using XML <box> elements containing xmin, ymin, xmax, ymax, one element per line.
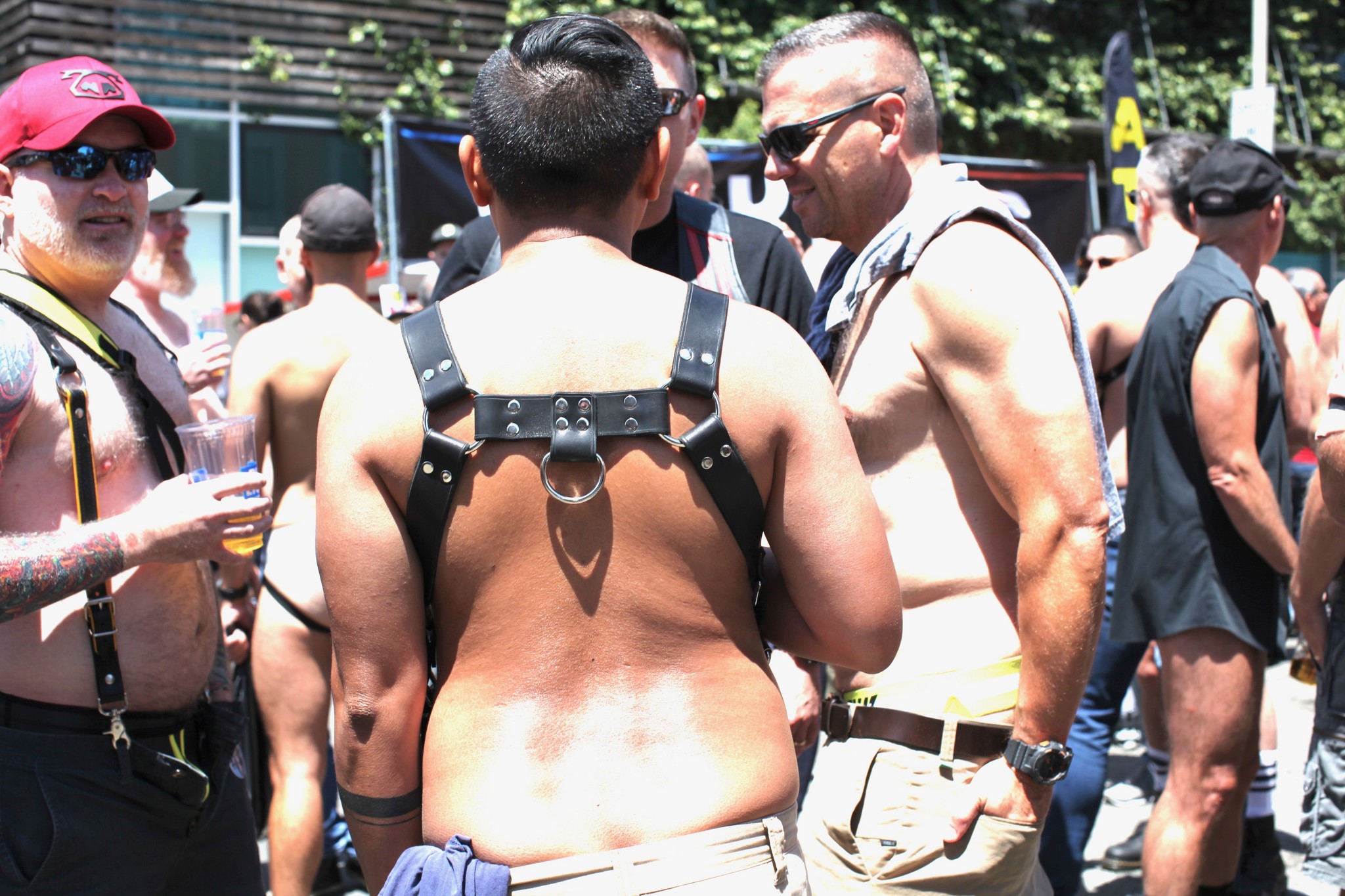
<box><xmin>1290</xmin><ymin>628</ymin><xmax>1318</xmax><ymax>685</ymax></box>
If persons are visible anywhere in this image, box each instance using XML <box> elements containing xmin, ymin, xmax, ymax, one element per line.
<box><xmin>750</xmin><ymin>10</ymin><xmax>1113</xmax><ymax>896</ymax></box>
<box><xmin>2</xmin><ymin>7</ymin><xmax>904</xmax><ymax>896</ymax></box>
<box><xmin>1037</xmin><ymin>123</ymin><xmax>1345</xmax><ymax>896</ymax></box>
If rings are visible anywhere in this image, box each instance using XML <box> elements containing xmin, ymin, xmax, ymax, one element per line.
<box><xmin>792</xmin><ymin>740</ymin><xmax>807</xmax><ymax>746</ymax></box>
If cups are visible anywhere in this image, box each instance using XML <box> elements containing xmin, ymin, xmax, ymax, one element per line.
<box><xmin>188</xmin><ymin>303</ymin><xmax>227</xmax><ymax>378</ymax></box>
<box><xmin>174</xmin><ymin>413</ymin><xmax>264</xmax><ymax>554</ymax></box>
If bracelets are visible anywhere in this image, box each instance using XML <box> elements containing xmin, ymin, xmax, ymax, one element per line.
<box><xmin>217</xmin><ymin>582</ymin><xmax>250</xmax><ymax>600</ymax></box>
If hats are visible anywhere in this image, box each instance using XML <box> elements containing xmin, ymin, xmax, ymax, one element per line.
<box><xmin>1</xmin><ymin>55</ymin><xmax>177</xmax><ymax>162</ymax></box>
<box><xmin>297</xmin><ymin>183</ymin><xmax>377</xmax><ymax>251</ymax></box>
<box><xmin>1188</xmin><ymin>137</ymin><xmax>1300</xmax><ymax>217</ymax></box>
<box><xmin>144</xmin><ymin>168</ymin><xmax>207</xmax><ymax>214</ymax></box>
<box><xmin>429</xmin><ymin>223</ymin><xmax>463</xmax><ymax>251</ymax></box>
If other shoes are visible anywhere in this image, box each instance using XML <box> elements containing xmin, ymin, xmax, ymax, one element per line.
<box><xmin>346</xmin><ymin>859</ymin><xmax>366</xmax><ymax>887</ymax></box>
<box><xmin>1237</xmin><ymin>815</ymin><xmax>1288</xmax><ymax>896</ymax></box>
<box><xmin>1286</xmin><ymin>874</ymin><xmax>1341</xmax><ymax>896</ymax></box>
<box><xmin>309</xmin><ymin>857</ymin><xmax>343</xmax><ymax>896</ymax></box>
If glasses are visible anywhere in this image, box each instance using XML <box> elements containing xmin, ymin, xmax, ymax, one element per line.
<box><xmin>5</xmin><ymin>139</ymin><xmax>158</xmax><ymax>182</ymax></box>
<box><xmin>658</xmin><ymin>88</ymin><xmax>694</xmax><ymax>116</ymax></box>
<box><xmin>1075</xmin><ymin>257</ymin><xmax>1124</xmax><ymax>269</ymax></box>
<box><xmin>757</xmin><ymin>85</ymin><xmax>907</xmax><ymax>161</ymax></box>
<box><xmin>1127</xmin><ymin>189</ymin><xmax>1142</xmax><ymax>204</ymax></box>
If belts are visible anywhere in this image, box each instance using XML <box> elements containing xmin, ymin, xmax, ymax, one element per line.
<box><xmin>821</xmin><ymin>697</ymin><xmax>1014</xmax><ymax>758</ymax></box>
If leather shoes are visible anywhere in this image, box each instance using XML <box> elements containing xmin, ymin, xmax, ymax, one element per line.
<box><xmin>1103</xmin><ymin>820</ymin><xmax>1149</xmax><ymax>871</ymax></box>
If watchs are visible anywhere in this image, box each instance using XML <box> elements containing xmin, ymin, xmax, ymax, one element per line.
<box><xmin>999</xmin><ymin>735</ymin><xmax>1074</xmax><ymax>785</ymax></box>
